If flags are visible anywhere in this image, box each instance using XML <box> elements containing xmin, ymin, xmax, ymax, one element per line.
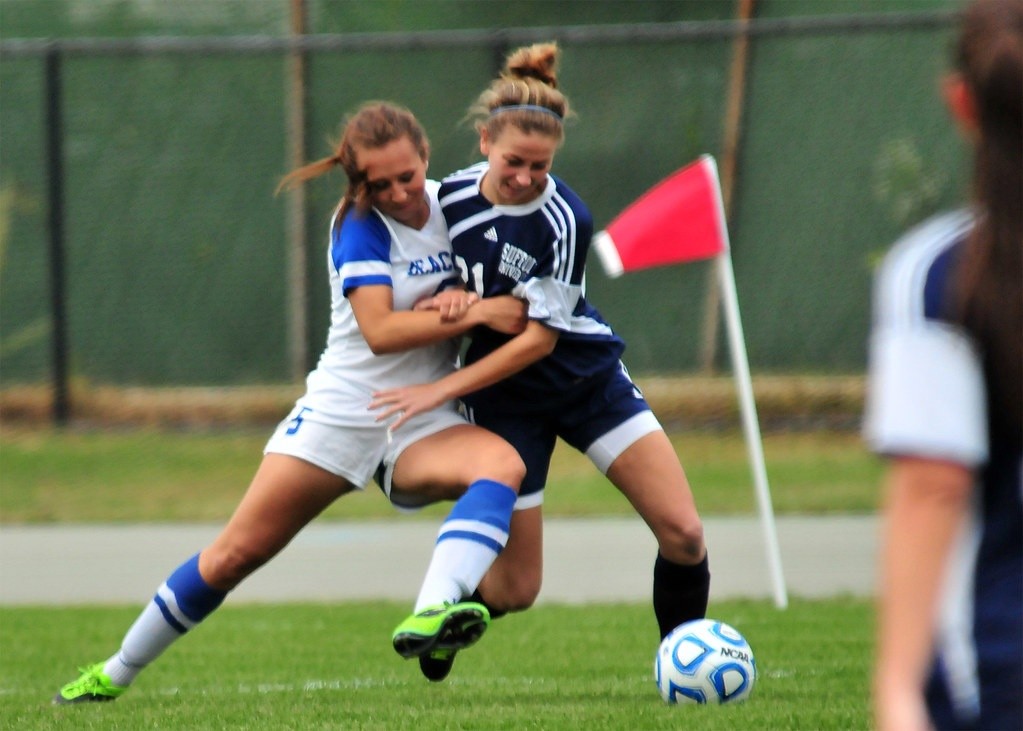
<box><xmin>591</xmin><ymin>157</ymin><xmax>725</xmax><ymax>278</ymax></box>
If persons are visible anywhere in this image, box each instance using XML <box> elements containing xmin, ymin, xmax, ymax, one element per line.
<box><xmin>859</xmin><ymin>0</ymin><xmax>1023</xmax><ymax>730</ymax></box>
<box><xmin>365</xmin><ymin>42</ymin><xmax>709</xmax><ymax>681</ymax></box>
<box><xmin>55</xmin><ymin>97</ymin><xmax>528</xmax><ymax>704</ymax></box>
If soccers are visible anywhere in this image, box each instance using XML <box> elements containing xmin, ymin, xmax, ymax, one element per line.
<box><xmin>654</xmin><ymin>618</ymin><xmax>754</xmax><ymax>708</ymax></box>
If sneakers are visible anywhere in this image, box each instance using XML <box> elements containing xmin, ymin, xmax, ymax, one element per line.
<box><xmin>51</xmin><ymin>661</ymin><xmax>126</xmax><ymax>707</ymax></box>
<box><xmin>393</xmin><ymin>601</ymin><xmax>491</xmax><ymax>659</ymax></box>
<box><xmin>420</xmin><ymin>640</ymin><xmax>458</xmax><ymax>682</ymax></box>
